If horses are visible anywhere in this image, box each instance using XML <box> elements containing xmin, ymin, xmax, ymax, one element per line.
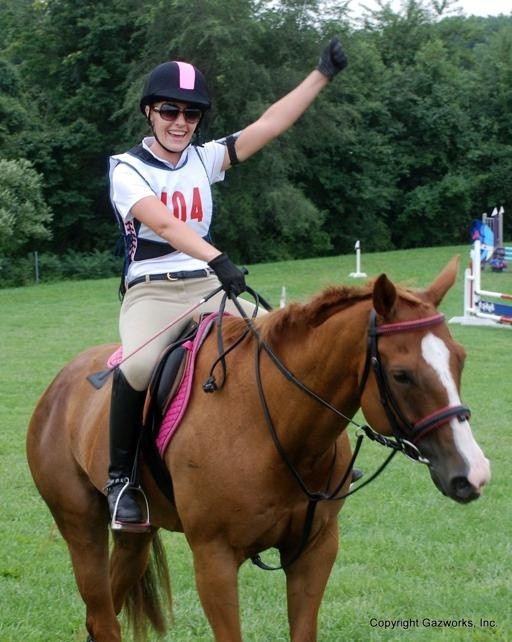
<box><xmin>26</xmin><ymin>253</ymin><xmax>492</xmax><ymax>641</ymax></box>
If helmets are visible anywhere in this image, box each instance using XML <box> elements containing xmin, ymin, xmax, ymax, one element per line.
<box><xmin>139</xmin><ymin>59</ymin><xmax>212</xmax><ymax>110</ymax></box>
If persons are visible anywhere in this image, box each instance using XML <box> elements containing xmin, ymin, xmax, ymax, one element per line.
<box><xmin>105</xmin><ymin>34</ymin><xmax>347</xmax><ymax>523</ymax></box>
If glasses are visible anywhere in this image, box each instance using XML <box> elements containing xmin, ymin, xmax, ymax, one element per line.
<box><xmin>147</xmin><ymin>102</ymin><xmax>204</xmax><ymax>125</ymax></box>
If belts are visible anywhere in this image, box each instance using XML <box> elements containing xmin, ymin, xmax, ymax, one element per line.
<box><xmin>126</xmin><ymin>268</ymin><xmax>216</xmax><ymax>291</ymax></box>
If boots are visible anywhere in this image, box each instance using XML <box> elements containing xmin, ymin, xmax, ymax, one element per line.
<box><xmin>104</xmin><ymin>365</ymin><xmax>150</xmax><ymax>524</ymax></box>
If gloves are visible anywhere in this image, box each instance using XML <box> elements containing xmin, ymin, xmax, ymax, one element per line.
<box><xmin>207</xmin><ymin>250</ymin><xmax>249</xmax><ymax>301</ymax></box>
<box><xmin>313</xmin><ymin>36</ymin><xmax>349</xmax><ymax>85</ymax></box>
